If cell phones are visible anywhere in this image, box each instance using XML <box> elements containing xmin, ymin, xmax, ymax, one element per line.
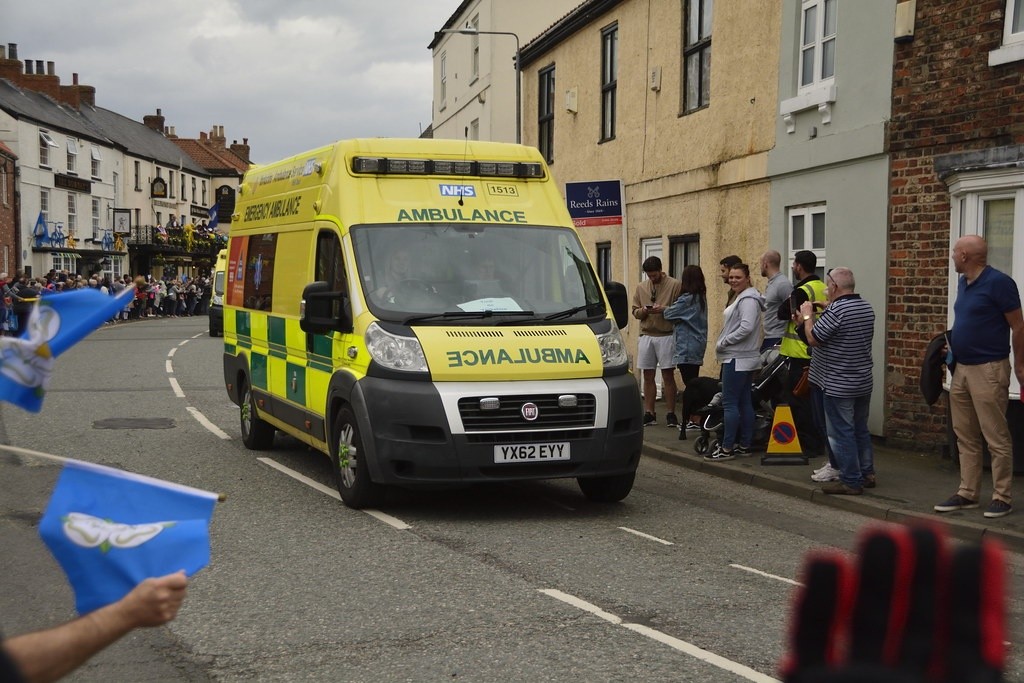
<box><xmin>813</xmin><ymin>304</ymin><xmax>817</xmax><ymax>312</ymax></box>
<box><xmin>789</xmin><ymin>295</ymin><xmax>797</xmax><ymax>314</ymax></box>
<box><xmin>646</xmin><ymin>305</ymin><xmax>653</xmax><ymax>309</ymax></box>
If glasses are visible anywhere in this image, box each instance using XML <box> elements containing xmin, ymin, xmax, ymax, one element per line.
<box><xmin>651</xmin><ymin>288</ymin><xmax>656</xmax><ymax>303</ymax></box>
<box><xmin>827</xmin><ymin>269</ymin><xmax>839</xmax><ymax>288</ymax></box>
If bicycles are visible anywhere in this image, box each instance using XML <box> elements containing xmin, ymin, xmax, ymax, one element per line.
<box><xmin>98</xmin><ymin>228</ymin><xmax>127</xmax><ymax>252</ymax></box>
<box><xmin>44</xmin><ymin>220</ymin><xmax>65</xmax><ymax>248</ymax></box>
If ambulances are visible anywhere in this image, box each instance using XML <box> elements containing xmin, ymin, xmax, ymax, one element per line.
<box><xmin>209</xmin><ymin>249</ymin><xmax>227</xmax><ymax>337</ymax></box>
<box><xmin>220</xmin><ymin>137</ymin><xmax>643</xmax><ymax>510</ymax></box>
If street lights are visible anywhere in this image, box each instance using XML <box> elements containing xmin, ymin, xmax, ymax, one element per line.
<box><xmin>457</xmin><ymin>24</ymin><xmax>523</xmax><ymax>144</ymax></box>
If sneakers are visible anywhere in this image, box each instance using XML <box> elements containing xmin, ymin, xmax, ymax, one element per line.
<box><xmin>643</xmin><ymin>411</ymin><xmax>658</xmax><ymax>427</ymax></box>
<box><xmin>679</xmin><ymin>420</ymin><xmax>701</xmax><ymax>431</ymax></box>
<box><xmin>934</xmin><ymin>494</ymin><xmax>980</xmax><ymax>511</ymax></box>
<box><xmin>861</xmin><ymin>474</ymin><xmax>877</xmax><ymax>488</ymax></box>
<box><xmin>811</xmin><ymin>468</ymin><xmax>842</xmax><ymax>481</ymax></box>
<box><xmin>666</xmin><ymin>412</ymin><xmax>678</xmax><ymax>427</ymax></box>
<box><xmin>821</xmin><ymin>480</ymin><xmax>862</xmax><ymax>495</ymax></box>
<box><xmin>732</xmin><ymin>445</ymin><xmax>752</xmax><ymax>457</ymax></box>
<box><xmin>704</xmin><ymin>447</ymin><xmax>735</xmax><ymax>461</ymax></box>
<box><xmin>814</xmin><ymin>462</ymin><xmax>831</xmax><ymax>474</ymax></box>
<box><xmin>984</xmin><ymin>499</ymin><xmax>1012</xmax><ymax>517</ymax></box>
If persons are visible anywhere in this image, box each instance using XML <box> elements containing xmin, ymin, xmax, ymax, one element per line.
<box><xmin>664</xmin><ymin>264</ymin><xmax>710</xmax><ymax>441</ymax></box>
<box><xmin>690</xmin><ymin>250</ymin><xmax>877</xmax><ymax>495</ymax></box>
<box><xmin>149</xmin><ymin>217</ymin><xmax>229</xmax><ymax>246</ymax></box>
<box><xmin>0</xmin><ymin>268</ymin><xmax>219</xmax><ymax>331</ymax></box>
<box><xmin>777</xmin><ymin>525</ymin><xmax>1003</xmax><ymax>683</ymax></box>
<box><xmin>459</xmin><ymin>251</ymin><xmax>516</xmax><ymax>298</ymax></box>
<box><xmin>935</xmin><ymin>235</ymin><xmax>1024</xmax><ymax>517</ymax></box>
<box><xmin>0</xmin><ymin>567</ymin><xmax>186</xmax><ymax>683</ymax></box>
<box><xmin>371</xmin><ymin>245</ymin><xmax>440</xmax><ymax>307</ymax></box>
<box><xmin>632</xmin><ymin>256</ymin><xmax>682</xmax><ymax>427</ymax></box>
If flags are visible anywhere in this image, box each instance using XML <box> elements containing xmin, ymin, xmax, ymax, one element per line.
<box><xmin>41</xmin><ymin>460</ymin><xmax>216</xmax><ymax>617</ymax></box>
<box><xmin>34</xmin><ymin>210</ymin><xmax>50</xmax><ymax>247</ymax></box>
<box><xmin>208</xmin><ymin>203</ymin><xmax>219</xmax><ymax>230</ymax></box>
<box><xmin>0</xmin><ymin>283</ymin><xmax>137</xmax><ymax>413</ymax></box>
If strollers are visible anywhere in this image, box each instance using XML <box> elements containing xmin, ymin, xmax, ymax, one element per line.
<box><xmin>692</xmin><ymin>339</ymin><xmax>792</xmax><ymax>456</ymax></box>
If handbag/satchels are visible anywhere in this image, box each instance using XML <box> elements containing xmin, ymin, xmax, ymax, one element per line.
<box><xmin>792</xmin><ymin>370</ymin><xmax>810</xmax><ymax>402</ymax></box>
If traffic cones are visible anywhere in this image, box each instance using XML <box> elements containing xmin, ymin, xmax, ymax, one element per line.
<box><xmin>761</xmin><ymin>405</ymin><xmax>810</xmax><ymax>465</ymax></box>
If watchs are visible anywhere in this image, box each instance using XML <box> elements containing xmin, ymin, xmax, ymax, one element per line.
<box><xmin>803</xmin><ymin>315</ymin><xmax>812</xmax><ymax>321</ymax></box>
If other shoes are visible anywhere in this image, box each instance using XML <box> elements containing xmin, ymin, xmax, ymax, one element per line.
<box><xmin>696</xmin><ymin>402</ymin><xmax>719</xmax><ymax>412</ymax></box>
